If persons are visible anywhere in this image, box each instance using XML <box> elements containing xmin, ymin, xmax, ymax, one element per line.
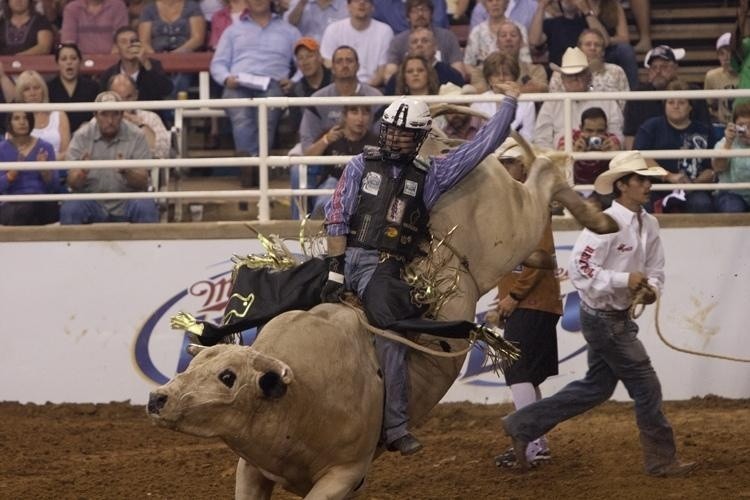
<box><xmin>208</xmin><ymin>0</ymin><xmax>467</xmax><ymax>219</ymax></box>
<box><xmin>463</xmin><ymin>0</ymin><xmax>749</xmax><ymax>212</ymax></box>
<box><xmin>484</xmin><ymin>216</ymin><xmax>566</xmax><ymax>474</ymax></box>
<box><xmin>321</xmin><ymin>77</ymin><xmax>522</xmax><ymax>457</ymax></box>
<box><xmin>1</xmin><ymin>1</ymin><xmax>206</xmax><ymax>226</ymax></box>
<box><xmin>499</xmin><ymin>148</ymin><xmax>700</xmax><ymax>481</ymax></box>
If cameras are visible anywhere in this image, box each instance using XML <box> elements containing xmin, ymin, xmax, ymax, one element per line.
<box><xmin>735</xmin><ymin>125</ymin><xmax>744</xmax><ymax>137</ymax></box>
<box><xmin>587</xmin><ymin>135</ymin><xmax>603</xmax><ymax>148</ymax></box>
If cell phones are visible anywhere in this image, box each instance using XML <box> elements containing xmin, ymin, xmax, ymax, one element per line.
<box><xmin>129</xmin><ymin>35</ymin><xmax>139</xmax><ymax>46</ymax></box>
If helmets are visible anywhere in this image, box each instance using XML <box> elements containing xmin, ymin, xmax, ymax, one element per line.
<box><xmin>493</xmin><ymin>136</ymin><xmax>526</xmax><ymax>161</ymax></box>
<box><xmin>381</xmin><ymin>97</ymin><xmax>433</xmax><ymax>133</ymax></box>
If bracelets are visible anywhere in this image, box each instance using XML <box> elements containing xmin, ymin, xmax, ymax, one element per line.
<box><xmin>510</xmin><ymin>291</ymin><xmax>522</xmax><ymax>301</ymax></box>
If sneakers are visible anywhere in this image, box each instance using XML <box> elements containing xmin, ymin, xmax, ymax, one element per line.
<box><xmin>492</xmin><ymin>435</ymin><xmax>551</xmax><ymax>466</ymax></box>
<box><xmin>494</xmin><ymin>445</ymin><xmax>553</xmax><ymax>466</ymax></box>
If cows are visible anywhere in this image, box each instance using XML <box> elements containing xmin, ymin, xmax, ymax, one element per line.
<box><xmin>147</xmin><ymin>102</ymin><xmax>620</xmax><ymax>499</ymax></box>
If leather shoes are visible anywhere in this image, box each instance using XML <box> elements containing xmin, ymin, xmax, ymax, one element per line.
<box><xmin>648</xmin><ymin>460</ymin><xmax>698</xmax><ymax>480</ymax></box>
<box><xmin>501</xmin><ymin>416</ymin><xmax>532</xmax><ymax>469</ymax></box>
<box><xmin>385</xmin><ymin>432</ymin><xmax>424</xmax><ymax>457</ymax></box>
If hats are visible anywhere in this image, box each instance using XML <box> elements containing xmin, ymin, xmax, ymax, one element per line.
<box><xmin>647</xmin><ymin>45</ymin><xmax>677</xmax><ymax>65</ymax></box>
<box><xmin>548</xmin><ymin>46</ymin><xmax>605</xmax><ymax>77</ymax></box>
<box><xmin>294</xmin><ymin>37</ymin><xmax>320</xmax><ymax>55</ymax></box>
<box><xmin>94</xmin><ymin>91</ymin><xmax>123</xmax><ymax>101</ymax></box>
<box><xmin>716</xmin><ymin>32</ymin><xmax>732</xmax><ymax>51</ymax></box>
<box><xmin>594</xmin><ymin>150</ymin><xmax>669</xmax><ymax>196</ymax></box>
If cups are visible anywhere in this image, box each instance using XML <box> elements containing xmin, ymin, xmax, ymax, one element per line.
<box><xmin>190</xmin><ymin>204</ymin><xmax>204</xmax><ymax>222</ymax></box>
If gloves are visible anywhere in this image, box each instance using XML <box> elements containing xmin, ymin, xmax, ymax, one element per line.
<box><xmin>319</xmin><ymin>253</ymin><xmax>347</xmax><ymax>304</ymax></box>
